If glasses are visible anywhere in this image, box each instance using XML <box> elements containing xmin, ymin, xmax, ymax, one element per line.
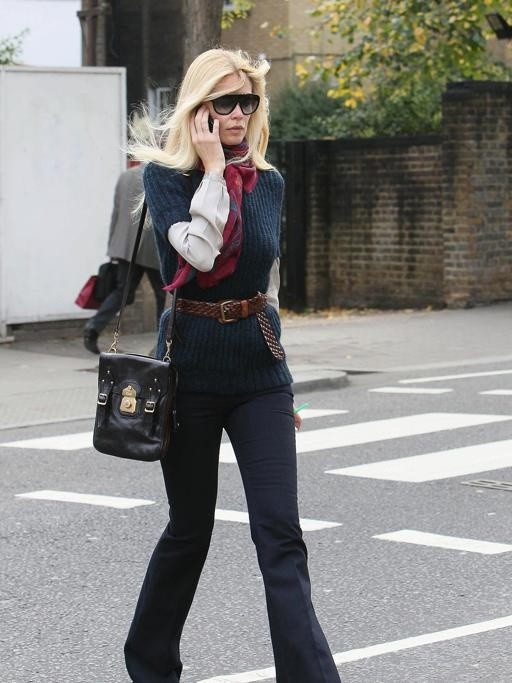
<box><xmin>210</xmin><ymin>94</ymin><xmax>260</xmax><ymax>115</ymax></box>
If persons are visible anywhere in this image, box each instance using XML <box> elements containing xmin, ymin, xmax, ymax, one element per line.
<box><xmin>80</xmin><ymin>161</ymin><xmax>167</xmax><ymax>354</ymax></box>
<box><xmin>124</xmin><ymin>46</ymin><xmax>343</xmax><ymax>682</ymax></box>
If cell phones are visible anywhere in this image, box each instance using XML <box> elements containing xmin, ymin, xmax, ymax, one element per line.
<box><xmin>196</xmin><ymin>104</ymin><xmax>214</xmax><ymax>132</ymax></box>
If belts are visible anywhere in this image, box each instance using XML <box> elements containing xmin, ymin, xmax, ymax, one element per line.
<box><xmin>175</xmin><ymin>290</ymin><xmax>285</xmax><ymax>361</ymax></box>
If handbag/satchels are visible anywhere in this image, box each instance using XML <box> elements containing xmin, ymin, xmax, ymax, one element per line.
<box><xmin>93</xmin><ymin>351</ymin><xmax>180</xmax><ymax>461</ymax></box>
<box><xmin>75</xmin><ymin>276</ymin><xmax>101</xmax><ymax>309</ymax></box>
<box><xmin>94</xmin><ymin>257</ymin><xmax>116</xmax><ymax>305</ymax></box>
